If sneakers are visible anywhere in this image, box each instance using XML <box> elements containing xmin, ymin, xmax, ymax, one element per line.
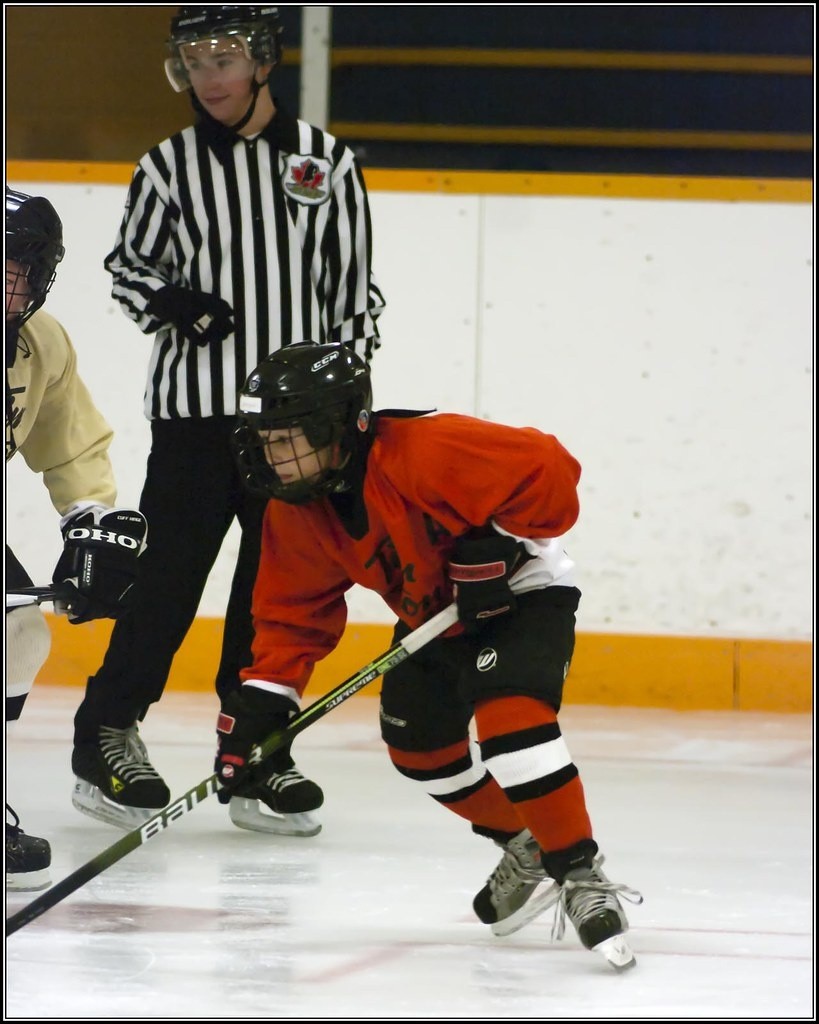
<box><xmin>6</xmin><ymin>805</ymin><xmax>53</xmax><ymax>905</ymax></box>
<box><xmin>229</xmin><ymin>764</ymin><xmax>323</xmax><ymax>836</ymax></box>
<box><xmin>471</xmin><ymin>821</ymin><xmax>563</xmax><ymax>937</ymax></box>
<box><xmin>70</xmin><ymin>676</ymin><xmax>169</xmax><ymax>833</ymax></box>
<box><xmin>540</xmin><ymin>839</ymin><xmax>645</xmax><ymax>970</ymax></box>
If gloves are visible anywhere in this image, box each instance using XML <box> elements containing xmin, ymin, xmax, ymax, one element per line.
<box><xmin>52</xmin><ymin>507</ymin><xmax>148</xmax><ymax>625</ymax></box>
<box><xmin>214</xmin><ymin>684</ymin><xmax>298</xmax><ymax>793</ymax></box>
<box><xmin>448</xmin><ymin>537</ymin><xmax>521</xmax><ymax>639</ymax></box>
<box><xmin>151</xmin><ymin>286</ymin><xmax>236</xmax><ymax>345</ymax></box>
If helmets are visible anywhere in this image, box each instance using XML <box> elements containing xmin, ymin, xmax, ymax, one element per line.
<box><xmin>5</xmin><ymin>188</ymin><xmax>66</xmax><ymax>369</ymax></box>
<box><xmin>171</xmin><ymin>6</ymin><xmax>284</xmax><ymax>88</ymax></box>
<box><xmin>231</xmin><ymin>341</ymin><xmax>372</xmax><ymax>504</ymax></box>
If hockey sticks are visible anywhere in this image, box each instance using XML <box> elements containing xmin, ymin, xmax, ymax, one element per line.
<box><xmin>6</xmin><ymin>575</ymin><xmax>79</xmax><ymax>610</ymax></box>
<box><xmin>5</xmin><ymin>557</ymin><xmax>555</xmax><ymax>936</ymax></box>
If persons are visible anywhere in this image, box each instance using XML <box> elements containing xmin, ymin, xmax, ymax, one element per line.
<box><xmin>5</xmin><ymin>187</ymin><xmax>148</xmax><ymax>874</ymax></box>
<box><xmin>71</xmin><ymin>6</ymin><xmax>386</xmax><ymax>837</ymax></box>
<box><xmin>220</xmin><ymin>341</ymin><xmax>643</xmax><ymax>966</ymax></box>
